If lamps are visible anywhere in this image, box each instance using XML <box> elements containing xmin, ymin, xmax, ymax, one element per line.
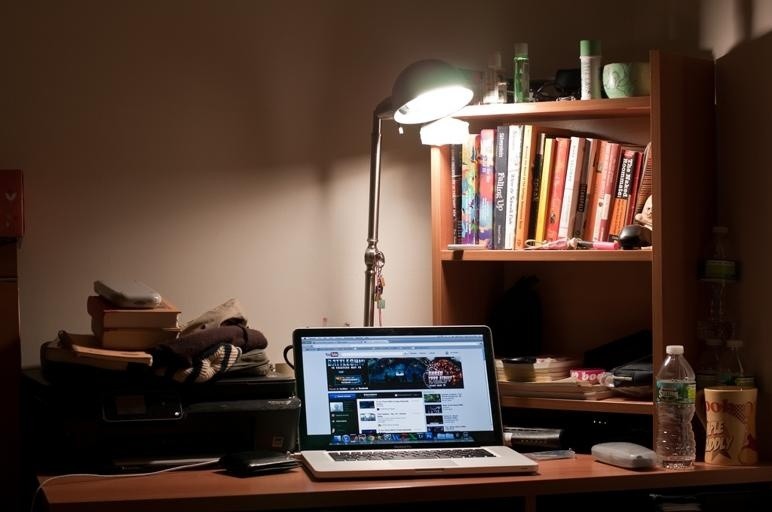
<box><xmin>364</xmin><ymin>59</ymin><xmax>475</xmax><ymax>327</ymax></box>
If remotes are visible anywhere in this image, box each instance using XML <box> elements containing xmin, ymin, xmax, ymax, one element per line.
<box><xmin>591</xmin><ymin>441</ymin><xmax>658</xmax><ymax>469</ymax></box>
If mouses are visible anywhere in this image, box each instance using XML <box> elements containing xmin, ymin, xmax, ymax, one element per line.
<box><xmin>617</xmin><ymin>225</ymin><xmax>652</xmax><ymax>247</ymax></box>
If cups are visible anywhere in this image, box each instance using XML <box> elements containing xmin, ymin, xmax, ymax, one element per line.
<box><xmin>694</xmin><ymin>386</ymin><xmax>759</xmax><ymax>467</ymax></box>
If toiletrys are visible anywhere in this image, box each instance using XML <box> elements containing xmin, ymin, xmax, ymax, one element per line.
<box><xmin>525</xmin><ymin>237</ymin><xmax>578</xmax><ymax>250</ymax></box>
<box><xmin>579</xmin><ymin>40</ymin><xmax>601</xmax><ymax>100</ymax></box>
<box><xmin>579</xmin><ymin>239</ymin><xmax>619</xmax><ymax>250</ymax></box>
<box><xmin>513</xmin><ymin>42</ymin><xmax>530</xmax><ymax>102</ymax></box>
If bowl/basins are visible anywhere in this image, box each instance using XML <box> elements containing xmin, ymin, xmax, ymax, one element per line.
<box><xmin>603</xmin><ymin>62</ymin><xmax>651</xmax><ymax>97</ymax></box>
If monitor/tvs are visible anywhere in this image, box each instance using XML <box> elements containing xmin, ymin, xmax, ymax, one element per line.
<box><xmin>291</xmin><ymin>324</ymin><xmax>540</xmax><ymax>479</ymax></box>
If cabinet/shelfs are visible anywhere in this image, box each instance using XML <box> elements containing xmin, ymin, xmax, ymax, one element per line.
<box><xmin>431</xmin><ymin>47</ymin><xmax>692</xmax><ymax>454</ymax></box>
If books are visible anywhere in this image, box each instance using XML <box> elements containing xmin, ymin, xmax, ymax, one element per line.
<box><xmin>87</xmin><ymin>295</ymin><xmax>184</xmax><ymax>329</ymax></box>
<box><xmin>449</xmin><ymin>123</ymin><xmax>651</xmax><ymax>250</ymax></box>
<box><xmin>90</xmin><ymin>316</ymin><xmax>182</xmax><ymax>350</ymax></box>
<box><xmin>58</xmin><ymin>330</ymin><xmax>153</xmax><ymax>365</ymax></box>
<box><xmin>48</xmin><ymin>338</ymin><xmax>128</xmax><ymax>369</ymax></box>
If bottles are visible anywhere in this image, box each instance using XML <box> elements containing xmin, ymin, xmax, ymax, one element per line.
<box><xmin>654</xmin><ymin>344</ymin><xmax>697</xmax><ymax>471</ymax></box>
<box><xmin>698</xmin><ymin>225</ymin><xmax>741</xmax><ymax>339</ymax></box>
<box><xmin>696</xmin><ymin>340</ymin><xmax>724</xmax><ymax>392</ymax></box>
<box><xmin>720</xmin><ymin>340</ymin><xmax>756</xmax><ymax>389</ymax></box>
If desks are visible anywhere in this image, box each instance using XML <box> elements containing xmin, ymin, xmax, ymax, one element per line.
<box><xmin>36</xmin><ymin>450</ymin><xmax>772</xmax><ymax>512</ymax></box>
